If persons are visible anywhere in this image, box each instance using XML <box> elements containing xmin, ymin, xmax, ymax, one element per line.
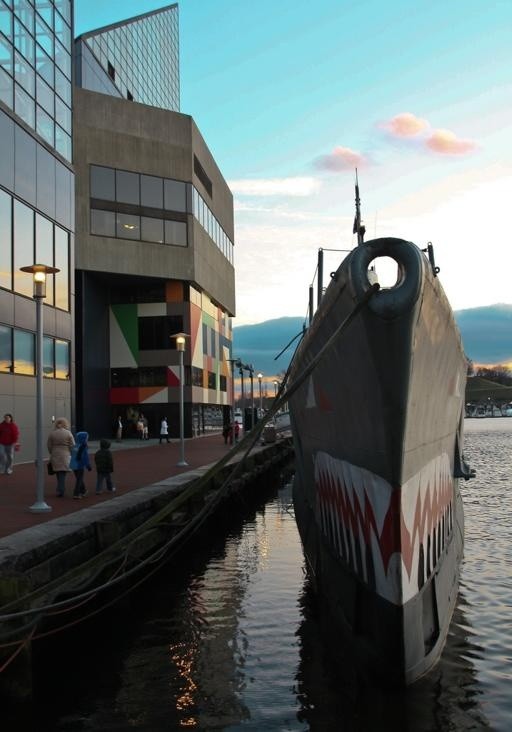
<box><xmin>234</xmin><ymin>422</ymin><xmax>239</xmax><ymax>443</ymax></box>
<box><xmin>115</xmin><ymin>414</ymin><xmax>171</xmax><ymax>443</ymax></box>
<box><xmin>94</xmin><ymin>441</ymin><xmax>116</xmax><ymax>494</ymax></box>
<box><xmin>229</xmin><ymin>423</ymin><xmax>234</xmax><ymax>445</ymax></box>
<box><xmin>48</xmin><ymin>417</ymin><xmax>75</xmax><ymax>498</ymax></box>
<box><xmin>71</xmin><ymin>431</ymin><xmax>92</xmax><ymax>500</ymax></box>
<box><xmin>0</xmin><ymin>413</ymin><xmax>20</xmax><ymax>475</ymax></box>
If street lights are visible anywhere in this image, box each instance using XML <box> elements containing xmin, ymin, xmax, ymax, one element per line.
<box><xmin>225</xmin><ymin>356</ymin><xmax>239</xmax><ymax>447</ymax></box>
<box><xmin>235</xmin><ymin>360</ymin><xmax>246</xmax><ymax>436</ymax></box>
<box><xmin>256</xmin><ymin>371</ymin><xmax>264</xmax><ymax>416</ymax></box>
<box><xmin>263</xmin><ymin>378</ymin><xmax>288</xmax><ymax>414</ymax></box>
<box><xmin>168</xmin><ymin>333</ymin><xmax>192</xmax><ymax>469</ymax></box>
<box><xmin>19</xmin><ymin>262</ymin><xmax>62</xmax><ymax>514</ymax></box>
<box><xmin>245</xmin><ymin>362</ymin><xmax>256</xmax><ymax>431</ymax></box>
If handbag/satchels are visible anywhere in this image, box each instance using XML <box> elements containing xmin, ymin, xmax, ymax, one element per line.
<box><xmin>47</xmin><ymin>461</ymin><xmax>53</xmax><ymax>476</ymax></box>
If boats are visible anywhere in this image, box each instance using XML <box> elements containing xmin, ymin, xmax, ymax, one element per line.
<box><xmin>283</xmin><ymin>164</ymin><xmax>479</xmax><ymax>690</ymax></box>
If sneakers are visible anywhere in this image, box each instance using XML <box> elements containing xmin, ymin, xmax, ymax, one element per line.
<box><xmin>54</xmin><ymin>487</ymin><xmax>115</xmax><ymax>499</ymax></box>
<box><xmin>7</xmin><ymin>468</ymin><xmax>12</xmax><ymax>474</ymax></box>
